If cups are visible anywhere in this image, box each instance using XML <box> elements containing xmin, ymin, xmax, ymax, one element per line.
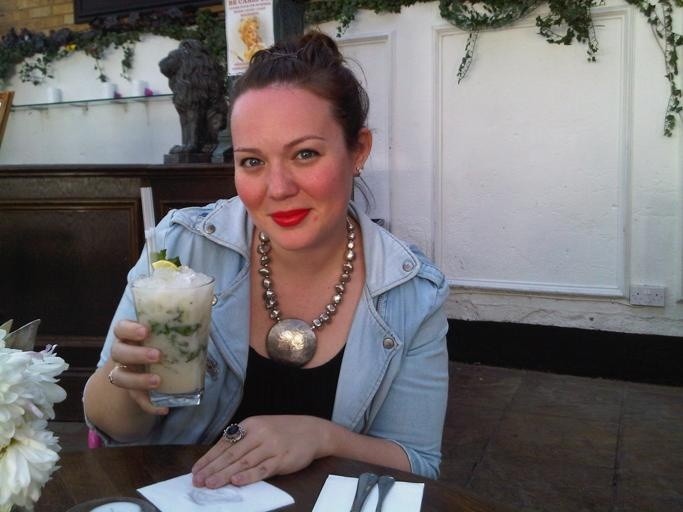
<box><xmin>131</xmin><ymin>273</ymin><xmax>215</xmax><ymax>407</ymax></box>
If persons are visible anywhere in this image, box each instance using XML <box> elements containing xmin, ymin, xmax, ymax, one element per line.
<box><xmin>80</xmin><ymin>29</ymin><xmax>451</xmax><ymax>489</ymax></box>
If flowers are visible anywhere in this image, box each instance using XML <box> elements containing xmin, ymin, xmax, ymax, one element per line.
<box><xmin>0</xmin><ymin>328</ymin><xmax>69</xmax><ymax>511</ymax></box>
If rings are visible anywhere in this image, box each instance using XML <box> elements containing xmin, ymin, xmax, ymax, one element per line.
<box><xmin>223</xmin><ymin>424</ymin><xmax>246</xmax><ymax>443</ymax></box>
<box><xmin>106</xmin><ymin>365</ymin><xmax>130</xmax><ymax>385</ymax></box>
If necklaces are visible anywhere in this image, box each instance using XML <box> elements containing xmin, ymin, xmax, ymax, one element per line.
<box><xmin>256</xmin><ymin>212</ymin><xmax>355</xmax><ymax>369</ymax></box>
